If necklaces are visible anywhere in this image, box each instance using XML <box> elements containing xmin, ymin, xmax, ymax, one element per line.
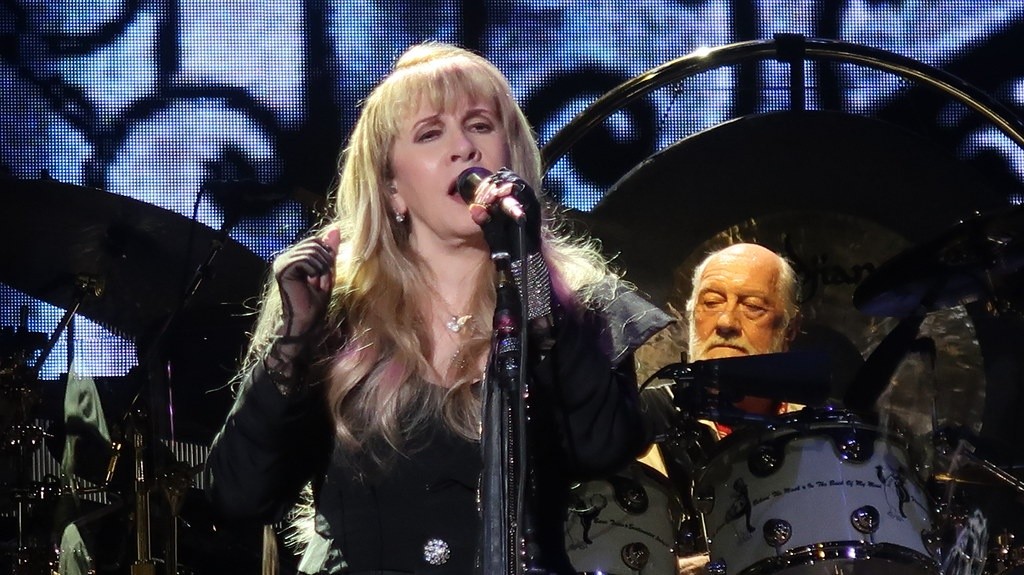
<box><xmin>424</xmin><ymin>278</ymin><xmax>484</xmax><ymax>372</ymax></box>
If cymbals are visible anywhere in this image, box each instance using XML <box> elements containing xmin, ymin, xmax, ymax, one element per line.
<box><xmin>1</xmin><ymin>177</ymin><xmax>269</xmax><ymax>349</ymax></box>
<box><xmin>55</xmin><ymin>370</ymin><xmax>233</xmax><ymax>447</ymax></box>
<box><xmin>44</xmin><ymin>419</ymin><xmax>181</xmax><ymax>491</ymax></box>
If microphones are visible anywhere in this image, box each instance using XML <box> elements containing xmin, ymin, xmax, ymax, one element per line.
<box><xmin>206</xmin><ymin>176</ymin><xmax>293</xmax><ymax>201</ymax></box>
<box><xmin>854</xmin><ymin>224</ymin><xmax>972</xmax><ymax>303</ymax></box>
<box><xmin>847</xmin><ymin>276</ymin><xmax>949</xmax><ymax>411</ymax></box>
<box><xmin>456</xmin><ymin>167</ymin><xmax>529</xmax><ymax>226</ymax></box>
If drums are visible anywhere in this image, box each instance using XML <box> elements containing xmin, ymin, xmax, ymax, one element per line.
<box><xmin>929</xmin><ymin>500</ymin><xmax>991</xmax><ymax>575</ymax></box>
<box><xmin>545</xmin><ymin>442</ymin><xmax>690</xmax><ymax>575</ymax></box>
<box><xmin>690</xmin><ymin>403</ymin><xmax>940</xmax><ymax>575</ymax></box>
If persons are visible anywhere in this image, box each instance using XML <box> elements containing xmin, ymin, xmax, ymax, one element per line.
<box><xmin>205</xmin><ymin>40</ymin><xmax>637</xmax><ymax>575</ymax></box>
<box><xmin>637</xmin><ymin>242</ymin><xmax>802</xmax><ymax>478</ymax></box>
